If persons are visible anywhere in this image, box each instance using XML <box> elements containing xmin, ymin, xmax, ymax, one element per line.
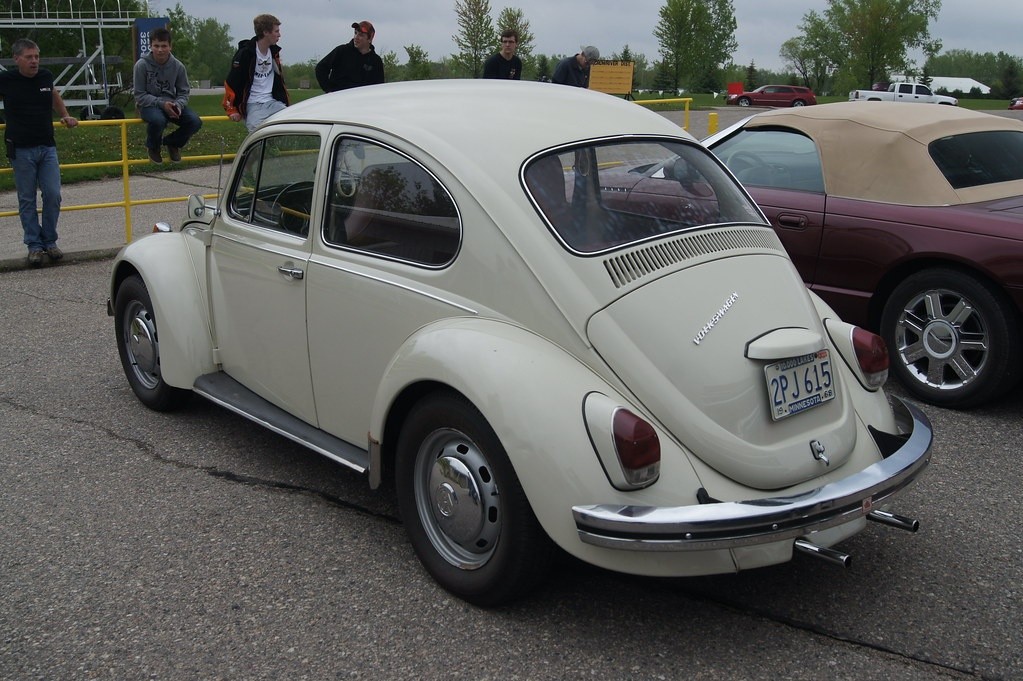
<box><xmin>132</xmin><ymin>28</ymin><xmax>203</xmax><ymax>166</ymax></box>
<box><xmin>0</xmin><ymin>39</ymin><xmax>80</xmax><ymax>265</ymax></box>
<box><xmin>551</xmin><ymin>45</ymin><xmax>600</xmax><ymax>91</ymax></box>
<box><xmin>483</xmin><ymin>30</ymin><xmax>522</xmax><ymax>82</ymax></box>
<box><xmin>315</xmin><ymin>21</ymin><xmax>389</xmax><ymax>94</ymax></box>
<box><xmin>223</xmin><ymin>13</ymin><xmax>290</xmax><ymax>131</ymax></box>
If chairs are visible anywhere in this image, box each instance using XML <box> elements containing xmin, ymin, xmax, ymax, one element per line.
<box><xmin>343</xmin><ymin>160</ymin><xmax>436</xmax><ymax>244</ymax></box>
<box><xmin>524</xmin><ymin>152</ymin><xmax>567</xmax><ymax>218</ymax></box>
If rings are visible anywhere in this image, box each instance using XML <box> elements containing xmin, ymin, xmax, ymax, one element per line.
<box><xmin>230</xmin><ymin>116</ymin><xmax>236</xmax><ymax>122</ymax></box>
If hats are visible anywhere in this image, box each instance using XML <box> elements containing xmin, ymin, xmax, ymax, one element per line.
<box><xmin>580</xmin><ymin>45</ymin><xmax>600</xmax><ymax>64</ymax></box>
<box><xmin>352</xmin><ymin>20</ymin><xmax>376</xmax><ymax>33</ymax></box>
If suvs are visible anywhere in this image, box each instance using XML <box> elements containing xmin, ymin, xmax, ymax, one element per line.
<box><xmin>726</xmin><ymin>84</ymin><xmax>818</xmax><ymax>109</ymax></box>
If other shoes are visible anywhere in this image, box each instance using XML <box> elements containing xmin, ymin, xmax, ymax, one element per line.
<box><xmin>167</xmin><ymin>144</ymin><xmax>181</xmax><ymax>163</ymax></box>
<box><xmin>27</xmin><ymin>250</ymin><xmax>44</xmax><ymax>263</ymax></box>
<box><xmin>144</xmin><ymin>144</ymin><xmax>163</xmax><ymax>165</ymax></box>
<box><xmin>45</xmin><ymin>246</ymin><xmax>63</xmax><ymax>259</ymax></box>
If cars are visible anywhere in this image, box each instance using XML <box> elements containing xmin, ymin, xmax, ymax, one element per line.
<box><xmin>106</xmin><ymin>77</ymin><xmax>935</xmax><ymax>613</ymax></box>
<box><xmin>565</xmin><ymin>100</ymin><xmax>1023</xmax><ymax>414</ymax></box>
<box><xmin>1008</xmin><ymin>97</ymin><xmax>1023</xmax><ymax>110</ymax></box>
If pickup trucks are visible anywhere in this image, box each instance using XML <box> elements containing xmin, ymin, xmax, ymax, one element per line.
<box><xmin>849</xmin><ymin>81</ymin><xmax>959</xmax><ymax>107</ymax></box>
